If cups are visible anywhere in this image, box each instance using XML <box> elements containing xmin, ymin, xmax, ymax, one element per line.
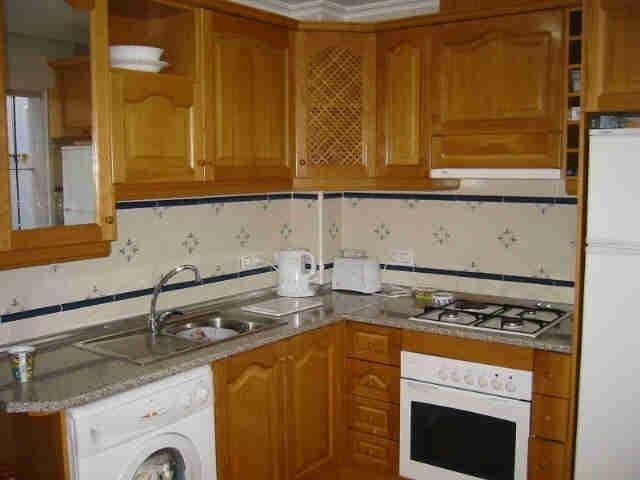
<box><xmin>6</xmin><ymin>344</ymin><xmax>38</xmax><ymax>385</ymax></box>
<box><xmin>569</xmin><ymin>69</ymin><xmax>581</xmax><ymax>92</ymax></box>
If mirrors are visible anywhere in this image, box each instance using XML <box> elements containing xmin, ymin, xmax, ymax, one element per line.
<box><xmin>1</xmin><ymin>1</ymin><xmax>118</xmax><ymax>252</ymax></box>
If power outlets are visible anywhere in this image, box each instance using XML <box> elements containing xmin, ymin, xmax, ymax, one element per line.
<box><xmin>390</xmin><ymin>251</ymin><xmax>414</xmax><ymax>267</ymax></box>
<box><xmin>240</xmin><ymin>254</ymin><xmax>253</xmax><ymax>272</ymax></box>
<box><xmin>255</xmin><ymin>251</ymin><xmax>267</xmax><ymax>267</ymax></box>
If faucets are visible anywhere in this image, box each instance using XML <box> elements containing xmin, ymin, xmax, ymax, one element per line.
<box><xmin>146</xmin><ymin>264</ymin><xmax>203</xmax><ymax>336</ymax></box>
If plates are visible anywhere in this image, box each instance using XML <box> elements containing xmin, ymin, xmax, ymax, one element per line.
<box><xmin>109</xmin><ymin>42</ymin><xmax>174</xmax><ymax>73</ymax></box>
<box><xmin>172</xmin><ymin>325</ymin><xmax>241</xmax><ymax>346</ymax></box>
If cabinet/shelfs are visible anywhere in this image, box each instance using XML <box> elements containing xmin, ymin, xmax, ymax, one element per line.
<box><xmin>563</xmin><ymin>1</ymin><xmax>640</xmax><ymax>196</ymax></box>
<box><xmin>526</xmin><ymin>350</ymin><xmax>576</xmax><ymax>480</ymax></box>
<box><xmin>345</xmin><ymin>320</ymin><xmax>402</xmax><ymax>480</ymax></box>
<box><xmin>377</xmin><ymin>14</ymin><xmax>460</xmax><ymax>192</ymax></box>
<box><xmin>294</xmin><ymin>20</ymin><xmax>377</xmax><ymax>193</ymax></box>
<box><xmin>47</xmin><ymin>56</ymin><xmax>92</xmax><ymax>146</ymax></box>
<box><xmin>107</xmin><ymin>0</ymin><xmax>294</xmax><ymax>208</ymax></box>
<box><xmin>210</xmin><ymin>321</ymin><xmax>345</xmax><ymax>480</ymax></box>
<box><xmin>430</xmin><ymin>1</ymin><xmax>564</xmax><ymax>183</ymax></box>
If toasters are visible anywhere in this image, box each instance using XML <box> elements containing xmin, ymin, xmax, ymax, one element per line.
<box><xmin>330</xmin><ymin>248</ymin><xmax>383</xmax><ymax>297</ymax></box>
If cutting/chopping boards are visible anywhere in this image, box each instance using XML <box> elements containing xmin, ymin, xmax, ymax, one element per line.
<box><xmin>240</xmin><ymin>295</ymin><xmax>323</xmax><ymax>318</ymax></box>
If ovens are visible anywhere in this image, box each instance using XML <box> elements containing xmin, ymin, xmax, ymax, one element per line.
<box><xmin>399</xmin><ymin>352</ymin><xmax>533</xmax><ymax>480</ymax></box>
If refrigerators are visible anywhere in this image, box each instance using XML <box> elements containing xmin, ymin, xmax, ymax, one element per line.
<box><xmin>573</xmin><ymin>127</ymin><xmax>640</xmax><ymax>480</ymax></box>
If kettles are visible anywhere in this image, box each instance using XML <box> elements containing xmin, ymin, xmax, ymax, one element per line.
<box><xmin>273</xmin><ymin>245</ymin><xmax>318</xmax><ymax>298</ymax></box>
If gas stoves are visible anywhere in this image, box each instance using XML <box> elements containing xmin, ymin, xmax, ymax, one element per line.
<box><xmin>407</xmin><ymin>297</ymin><xmax>574</xmax><ymax>339</ymax></box>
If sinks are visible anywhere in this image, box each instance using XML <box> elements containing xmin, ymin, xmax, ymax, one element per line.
<box><xmin>164</xmin><ymin>302</ymin><xmax>290</xmax><ymax>346</ymax></box>
